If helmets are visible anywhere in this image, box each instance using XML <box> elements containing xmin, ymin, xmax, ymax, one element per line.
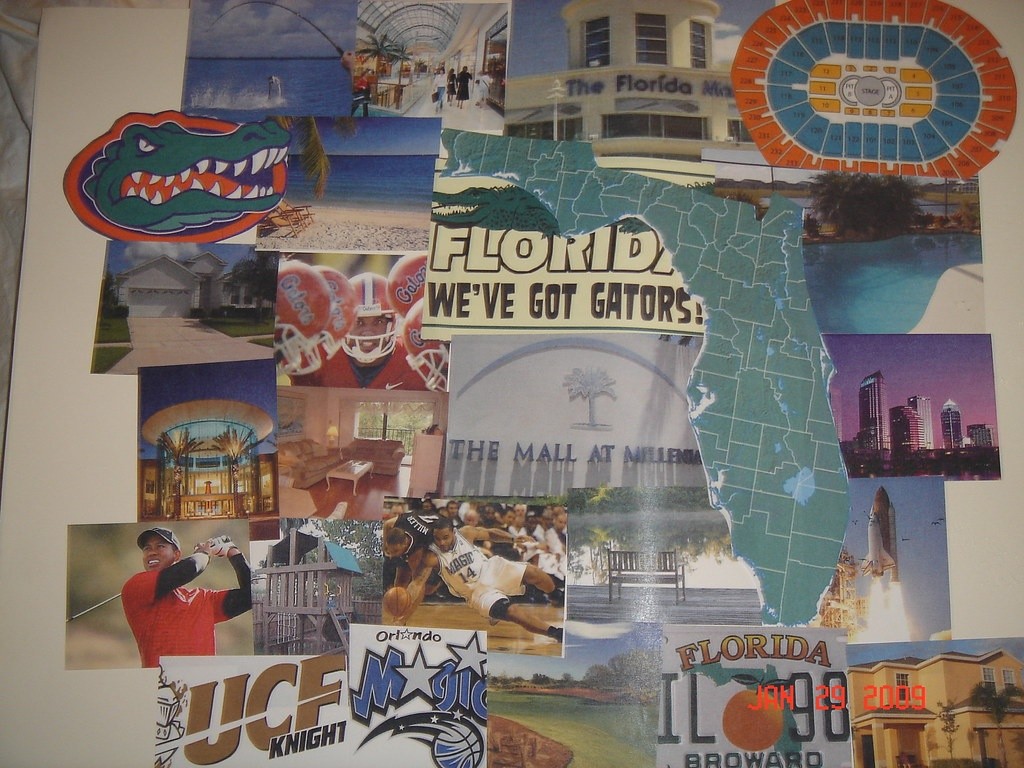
<box><xmin>388</xmin><ymin>255</ymin><xmax>429</xmax><ymax>318</ymax></box>
<box><xmin>274</xmin><ymin>261</ymin><xmax>332</xmax><ymax>364</ymax></box>
<box><xmin>339</xmin><ymin>272</ymin><xmax>398</xmax><ymax>363</ymax></box>
<box><xmin>403</xmin><ymin>297</ymin><xmax>450</xmax><ymax>392</ymax></box>
<box><xmin>281</xmin><ymin>265</ymin><xmax>356</xmax><ymax>376</ymax></box>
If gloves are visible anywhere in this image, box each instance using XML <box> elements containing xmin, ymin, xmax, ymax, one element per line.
<box><xmin>209</xmin><ymin>534</ymin><xmax>238</xmax><ymax>558</ymax></box>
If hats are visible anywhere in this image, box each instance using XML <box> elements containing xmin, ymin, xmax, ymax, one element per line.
<box><xmin>137</xmin><ymin>527</ymin><xmax>180</xmax><ymax>552</ymax></box>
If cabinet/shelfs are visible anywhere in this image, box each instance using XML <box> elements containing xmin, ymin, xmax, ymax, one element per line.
<box><xmin>409</xmin><ymin>433</ymin><xmax>445</xmax><ymax>498</ymax></box>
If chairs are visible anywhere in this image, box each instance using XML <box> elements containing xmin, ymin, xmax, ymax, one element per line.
<box><xmin>256</xmin><ymin>199</ymin><xmax>316</xmax><ymax>240</ymax></box>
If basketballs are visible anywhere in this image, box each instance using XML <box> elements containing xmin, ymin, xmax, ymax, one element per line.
<box><xmin>383</xmin><ymin>587</ymin><xmax>413</xmax><ymax>618</ymax></box>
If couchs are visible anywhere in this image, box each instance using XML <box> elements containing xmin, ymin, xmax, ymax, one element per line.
<box><xmin>276</xmin><ymin>438</ymin><xmax>340</xmax><ymax>489</ymax></box>
<box><xmin>346</xmin><ymin>439</ymin><xmax>405</xmax><ymax>476</ymax></box>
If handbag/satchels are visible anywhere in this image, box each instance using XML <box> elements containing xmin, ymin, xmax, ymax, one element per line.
<box><xmin>432</xmin><ymin>91</ymin><xmax>439</xmax><ymax>103</ymax></box>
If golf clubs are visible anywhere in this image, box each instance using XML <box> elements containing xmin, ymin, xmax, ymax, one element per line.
<box><xmin>67</xmin><ymin>536</ymin><xmax>229</xmax><ymax>624</ymax></box>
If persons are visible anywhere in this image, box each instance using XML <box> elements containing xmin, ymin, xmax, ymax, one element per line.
<box><xmin>383</xmin><ymin>498</ymin><xmax>566</xmax><ymax>641</ymax></box>
<box><xmin>276</xmin><ymin>251</ymin><xmax>450</xmax><ymax>392</ymax></box>
<box><xmin>121</xmin><ymin>527</ymin><xmax>251</xmax><ymax>666</ymax></box>
<box><xmin>431</xmin><ymin>65</ymin><xmax>490</xmax><ymax>113</ymax></box>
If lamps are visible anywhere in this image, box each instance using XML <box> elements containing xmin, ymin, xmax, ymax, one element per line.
<box><xmin>328</xmin><ymin>426</ymin><xmax>338</xmax><ymax>446</ymax></box>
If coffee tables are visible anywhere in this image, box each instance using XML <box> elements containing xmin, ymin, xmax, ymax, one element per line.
<box><xmin>325</xmin><ymin>459</ymin><xmax>376</xmax><ymax>496</ymax></box>
